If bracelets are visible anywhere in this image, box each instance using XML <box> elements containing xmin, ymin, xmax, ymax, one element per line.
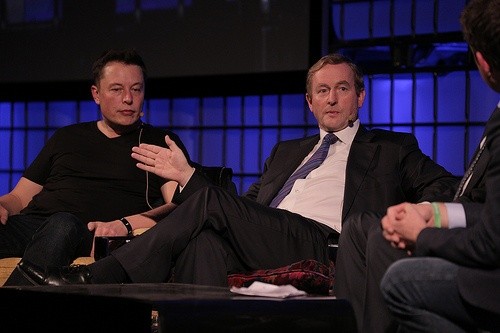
<box><xmin>433</xmin><ymin>202</ymin><xmax>441</xmax><ymax>229</ymax></box>
<box><xmin>118</xmin><ymin>218</ymin><xmax>133</xmax><ymax>237</ymax></box>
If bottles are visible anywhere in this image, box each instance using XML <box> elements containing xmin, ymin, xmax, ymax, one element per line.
<box><xmin>150</xmin><ymin>311</ymin><xmax>161</xmax><ymax>333</ymax></box>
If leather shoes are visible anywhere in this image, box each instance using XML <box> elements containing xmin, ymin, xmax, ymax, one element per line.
<box><xmin>16</xmin><ymin>260</ymin><xmax>93</xmax><ymax>286</ymax></box>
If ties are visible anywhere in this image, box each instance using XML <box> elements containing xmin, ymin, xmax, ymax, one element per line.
<box><xmin>269</xmin><ymin>133</ymin><xmax>337</xmax><ymax>208</ymax></box>
<box><xmin>453</xmin><ymin>139</ymin><xmax>488</xmax><ymax>201</ymax></box>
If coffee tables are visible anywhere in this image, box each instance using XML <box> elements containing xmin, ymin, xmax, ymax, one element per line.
<box><xmin>0</xmin><ymin>284</ymin><xmax>360</xmax><ymax>333</ymax></box>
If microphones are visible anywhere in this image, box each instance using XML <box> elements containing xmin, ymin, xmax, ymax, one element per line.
<box><xmin>348</xmin><ymin>120</ymin><xmax>354</xmax><ymax>127</ymax></box>
<box><xmin>139</xmin><ymin>112</ymin><xmax>144</xmax><ymax>127</ymax></box>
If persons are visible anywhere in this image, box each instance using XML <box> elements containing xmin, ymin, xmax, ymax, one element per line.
<box><xmin>0</xmin><ymin>48</ymin><xmax>191</xmax><ymax>286</ymax></box>
<box><xmin>18</xmin><ymin>54</ymin><xmax>461</xmax><ymax>287</ymax></box>
<box><xmin>331</xmin><ymin>0</ymin><xmax>500</xmax><ymax>333</ymax></box>
